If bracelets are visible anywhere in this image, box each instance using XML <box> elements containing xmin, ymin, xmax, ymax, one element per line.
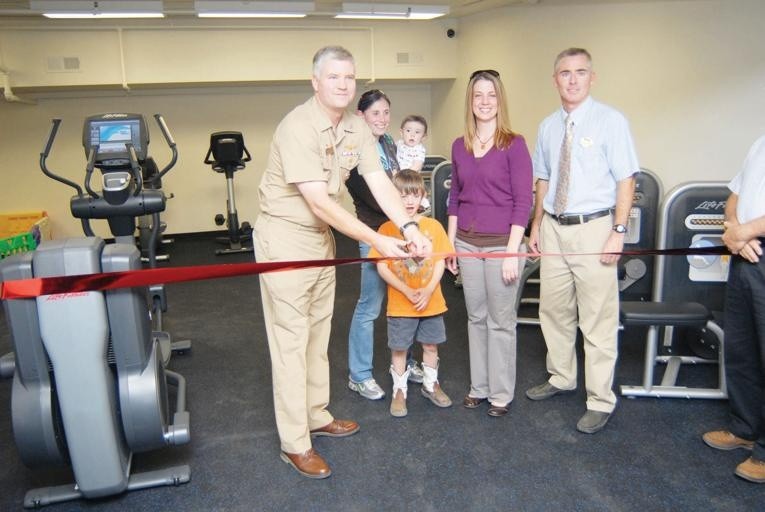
<box><xmin>400</xmin><ymin>221</ymin><xmax>419</xmax><ymax>236</ymax></box>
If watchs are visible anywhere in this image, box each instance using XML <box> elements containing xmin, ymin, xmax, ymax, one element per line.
<box><xmin>612</xmin><ymin>224</ymin><xmax>627</xmax><ymax>233</ymax></box>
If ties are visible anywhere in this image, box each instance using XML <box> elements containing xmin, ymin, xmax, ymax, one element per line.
<box><xmin>553</xmin><ymin>115</ymin><xmax>573</xmax><ymax>217</ymax></box>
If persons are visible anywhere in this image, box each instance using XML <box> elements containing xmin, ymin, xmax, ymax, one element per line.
<box><xmin>394</xmin><ymin>115</ymin><xmax>428</xmax><ymax>173</ymax></box>
<box><xmin>443</xmin><ymin>70</ymin><xmax>533</xmax><ymax>417</ymax></box>
<box><xmin>252</xmin><ymin>46</ymin><xmax>433</xmax><ymax>480</ymax></box>
<box><xmin>703</xmin><ymin>135</ymin><xmax>765</xmax><ymax>483</ymax></box>
<box><xmin>344</xmin><ymin>88</ymin><xmax>430</xmax><ymax>401</ymax></box>
<box><xmin>367</xmin><ymin>169</ymin><xmax>452</xmax><ymax>417</ymax></box>
<box><xmin>525</xmin><ymin>47</ymin><xmax>642</xmax><ymax>434</ymax></box>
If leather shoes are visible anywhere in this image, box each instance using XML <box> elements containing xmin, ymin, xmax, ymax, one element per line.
<box><xmin>464</xmin><ymin>394</ymin><xmax>486</xmax><ymax>409</ymax></box>
<box><xmin>280</xmin><ymin>448</ymin><xmax>331</xmax><ymax>479</ymax></box>
<box><xmin>311</xmin><ymin>418</ymin><xmax>361</xmax><ymax>437</ymax></box>
<box><xmin>488</xmin><ymin>404</ymin><xmax>511</xmax><ymax>417</ymax></box>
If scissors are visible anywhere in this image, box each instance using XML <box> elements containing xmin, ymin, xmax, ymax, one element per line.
<box><xmin>400</xmin><ymin>242</ymin><xmax>420</xmax><ymax>267</ymax></box>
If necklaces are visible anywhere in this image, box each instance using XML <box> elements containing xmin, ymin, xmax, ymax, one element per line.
<box><xmin>475</xmin><ymin>130</ymin><xmax>494</xmax><ymax>151</ymax></box>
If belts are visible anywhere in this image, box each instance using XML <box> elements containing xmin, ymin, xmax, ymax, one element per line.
<box><xmin>550</xmin><ymin>210</ymin><xmax>614</xmax><ymax>225</ymax></box>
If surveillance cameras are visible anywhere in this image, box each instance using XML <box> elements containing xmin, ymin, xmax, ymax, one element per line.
<box><xmin>446</xmin><ymin>27</ymin><xmax>457</xmax><ymax>40</ymax></box>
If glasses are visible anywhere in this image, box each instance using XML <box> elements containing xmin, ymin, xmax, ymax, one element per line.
<box><xmin>470</xmin><ymin>70</ymin><xmax>499</xmax><ymax>80</ymax></box>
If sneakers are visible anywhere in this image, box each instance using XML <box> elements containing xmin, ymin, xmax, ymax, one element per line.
<box><xmin>349</xmin><ymin>360</ymin><xmax>453</xmax><ymax>418</ymax></box>
<box><xmin>577</xmin><ymin>410</ymin><xmax>611</xmax><ymax>433</ymax></box>
<box><xmin>527</xmin><ymin>381</ymin><xmax>563</xmax><ymax>400</ymax></box>
<box><xmin>703</xmin><ymin>430</ymin><xmax>755</xmax><ymax>451</ymax></box>
<box><xmin>734</xmin><ymin>456</ymin><xmax>765</xmax><ymax>482</ymax></box>
